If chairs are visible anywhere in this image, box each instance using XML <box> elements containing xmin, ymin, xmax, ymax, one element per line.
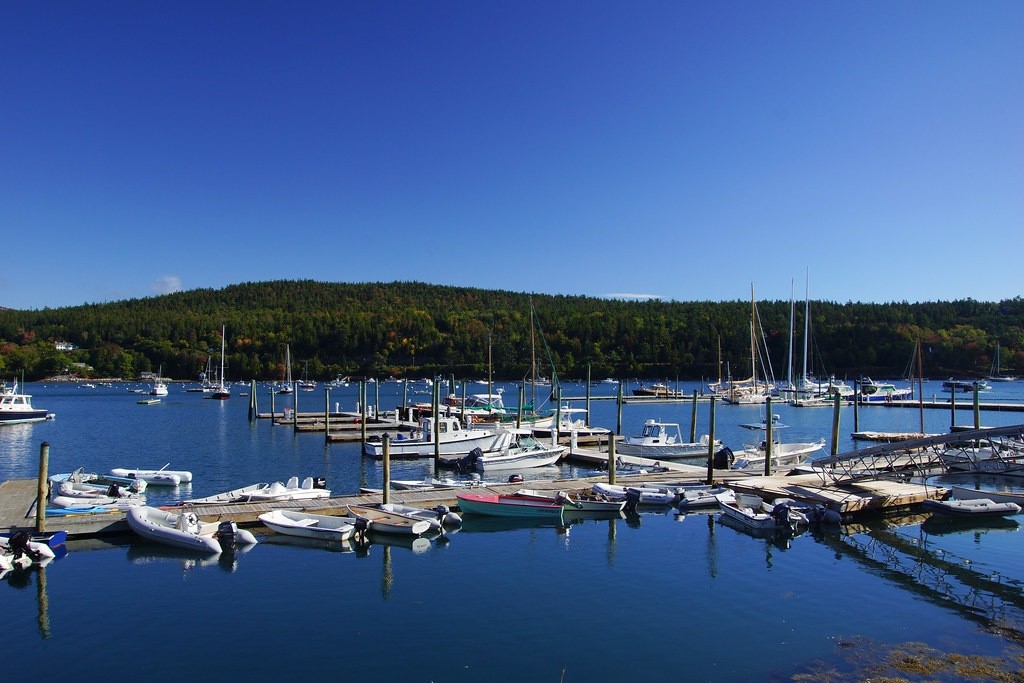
<box><xmin>286</xmin><ymin>477</ymin><xmax>314</xmax><ymax>490</ymax></box>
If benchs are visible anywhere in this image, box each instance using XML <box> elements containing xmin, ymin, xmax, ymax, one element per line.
<box><xmin>198</xmin><ymin>522</ymin><xmax>221</xmax><ymax>535</ymax></box>
<box><xmin>83</xmin><ymin>489</ymin><xmax>98</xmax><ymax>494</ymax></box>
<box><xmin>366</xmin><ymin>510</ymin><xmax>422</xmax><ymax>524</ymax></box>
<box><xmin>294</xmin><ymin>519</ymin><xmax>318</xmax><ymax>527</ymax></box>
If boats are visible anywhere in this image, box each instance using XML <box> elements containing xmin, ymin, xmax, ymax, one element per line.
<box><xmin>0</xmin><ymin>537</ymin><xmax>56</xmax><ymax>582</ymax></box>
<box><xmin>562</xmin><ymin>493</ymin><xmax>628</xmax><ymax>512</ymax></box>
<box><xmin>770</xmin><ymin>497</ymin><xmax>843</xmax><ymax>529</ymax></box>
<box><xmin>715</xmin><ymin>492</ymin><xmax>791</xmax><ymax>531</ymax></box>
<box><xmin>590</xmin><ymin>481</ymin><xmax>676</xmax><ymax>507</ymax></box>
<box><xmin>671</xmin><ymin>486</ymin><xmax>737</xmax><ymax>511</ymax></box>
<box><xmin>378</xmin><ymin>503</ymin><xmax>450</xmax><ymax>526</ymax></box>
<box><xmin>0</xmin><ymin>368</ymin><xmax>49</xmax><ymax>425</ymax></box>
<box><xmin>126</xmin><ymin>504</ymin><xmax>258</xmax><ymax>554</ymax></box>
<box><xmin>456</xmin><ymin>493</ymin><xmax>584</xmax><ymax>520</ymax></box>
<box><xmin>257</xmin><ymin>508</ymin><xmax>374</xmax><ymax>542</ymax></box>
<box><xmin>923</xmin><ymin>498</ymin><xmax>1023</xmax><ymax>519</ymax></box>
<box><xmin>345</xmin><ymin>504</ymin><xmax>442</xmax><ymax>536</ymax></box>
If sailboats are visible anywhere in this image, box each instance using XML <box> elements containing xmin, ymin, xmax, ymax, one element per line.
<box><xmin>41</xmin><ymin>269</ymin><xmax>1024</xmax><ymax>516</ymax></box>
